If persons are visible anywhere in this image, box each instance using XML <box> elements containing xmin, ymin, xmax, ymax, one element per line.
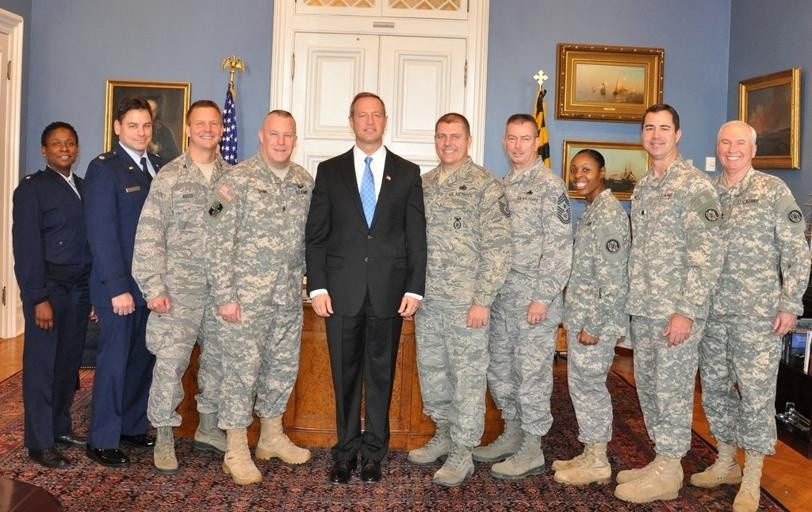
<box><xmin>130</xmin><ymin>100</ymin><xmax>233</xmax><ymax>477</ymax></box>
<box><xmin>613</xmin><ymin>103</ymin><xmax>725</xmax><ymax>504</ymax></box>
<box><xmin>304</xmin><ymin>92</ymin><xmax>428</xmax><ymax>485</ymax></box>
<box><xmin>472</xmin><ymin>113</ymin><xmax>574</xmax><ymax>481</ymax></box>
<box><xmin>406</xmin><ymin>112</ymin><xmax>513</xmax><ymax>488</ymax></box>
<box><xmin>688</xmin><ymin>121</ymin><xmax>812</xmax><ymax>512</ymax></box>
<box><xmin>83</xmin><ymin>94</ymin><xmax>166</xmax><ymax>469</ymax></box>
<box><xmin>144</xmin><ymin>93</ymin><xmax>179</xmax><ymax>164</ymax></box>
<box><xmin>551</xmin><ymin>149</ymin><xmax>632</xmax><ymax>487</ymax></box>
<box><xmin>202</xmin><ymin>109</ymin><xmax>315</xmax><ymax>486</ymax></box>
<box><xmin>12</xmin><ymin>121</ymin><xmax>99</xmax><ymax>468</ymax></box>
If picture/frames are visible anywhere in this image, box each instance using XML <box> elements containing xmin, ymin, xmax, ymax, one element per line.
<box><xmin>561</xmin><ymin>141</ymin><xmax>652</xmax><ymax>201</ymax></box>
<box><xmin>104</xmin><ymin>77</ymin><xmax>192</xmax><ymax>167</ymax></box>
<box><xmin>553</xmin><ymin>42</ymin><xmax>665</xmax><ymax>124</ymax></box>
<box><xmin>738</xmin><ymin>65</ymin><xmax>802</xmax><ymax>171</ymax></box>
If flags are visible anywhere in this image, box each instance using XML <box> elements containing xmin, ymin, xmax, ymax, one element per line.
<box><xmin>532</xmin><ymin>86</ymin><xmax>551</xmax><ymax>169</ymax></box>
<box><xmin>217</xmin><ymin>80</ymin><xmax>239</xmax><ymax>165</ymax></box>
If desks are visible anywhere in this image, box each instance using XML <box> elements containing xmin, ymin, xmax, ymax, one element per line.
<box><xmin>167</xmin><ymin>304</ymin><xmax>498</xmax><ymax>450</ymax></box>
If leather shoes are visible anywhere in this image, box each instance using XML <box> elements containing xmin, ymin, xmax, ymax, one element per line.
<box><xmin>329</xmin><ymin>454</ymin><xmax>357</xmax><ymax>483</ymax></box>
<box><xmin>360</xmin><ymin>457</ymin><xmax>381</xmax><ymax>483</ymax></box>
<box><xmin>29</xmin><ymin>429</ymin><xmax>153</xmax><ymax>467</ymax></box>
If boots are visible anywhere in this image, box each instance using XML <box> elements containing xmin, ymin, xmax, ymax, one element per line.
<box><xmin>154</xmin><ymin>425</ymin><xmax>178</xmax><ymax>474</ymax></box>
<box><xmin>255</xmin><ymin>415</ymin><xmax>311</xmax><ymax>464</ymax></box>
<box><xmin>408</xmin><ymin>425</ymin><xmax>545</xmax><ymax>488</ymax></box>
<box><xmin>733</xmin><ymin>450</ymin><xmax>764</xmax><ymax>512</ymax></box>
<box><xmin>550</xmin><ymin>444</ymin><xmax>612</xmax><ymax>484</ymax></box>
<box><xmin>192</xmin><ymin>412</ymin><xmax>226</xmax><ymax>458</ymax></box>
<box><xmin>690</xmin><ymin>439</ymin><xmax>742</xmax><ymax>488</ymax></box>
<box><xmin>223</xmin><ymin>428</ymin><xmax>262</xmax><ymax>486</ymax></box>
<box><xmin>614</xmin><ymin>453</ymin><xmax>684</xmax><ymax>503</ymax></box>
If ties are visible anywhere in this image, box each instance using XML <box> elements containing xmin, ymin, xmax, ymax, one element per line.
<box><xmin>140</xmin><ymin>158</ymin><xmax>154</xmax><ymax>182</ymax></box>
<box><xmin>360</xmin><ymin>157</ymin><xmax>376</xmax><ymax>227</ymax></box>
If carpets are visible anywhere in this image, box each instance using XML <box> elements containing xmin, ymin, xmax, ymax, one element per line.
<box><xmin>0</xmin><ymin>365</ymin><xmax>790</xmax><ymax>511</ymax></box>
